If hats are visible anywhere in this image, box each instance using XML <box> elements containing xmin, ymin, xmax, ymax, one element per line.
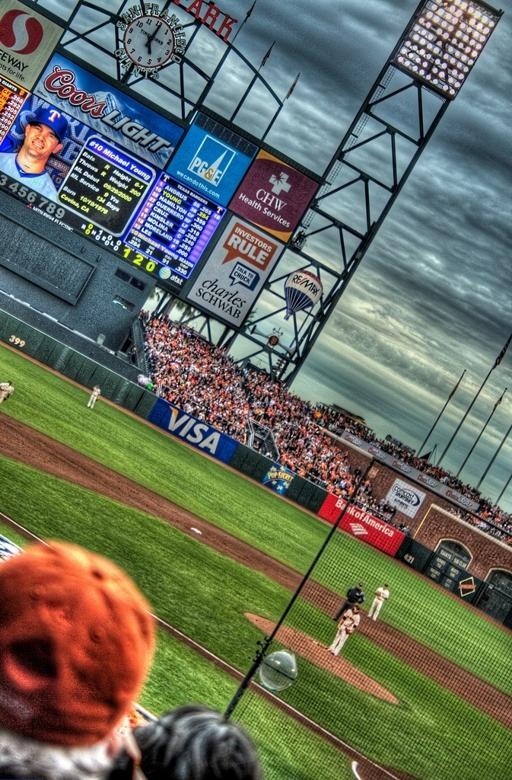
<box><xmin>28</xmin><ymin>107</ymin><xmax>69</xmax><ymax>143</ymax></box>
<box><xmin>0</xmin><ymin>541</ymin><xmax>157</xmax><ymax>751</ymax></box>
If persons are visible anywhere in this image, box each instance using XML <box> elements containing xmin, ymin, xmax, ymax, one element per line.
<box><xmin>325</xmin><ymin>604</ymin><xmax>362</xmax><ymax>656</ymax></box>
<box><xmin>140</xmin><ymin>305</ymin><xmax>511</xmax><ymax>549</ymax></box>
<box><xmin>0</xmin><ymin>105</ymin><xmax>70</xmax><ymax>205</ymax></box>
<box><xmin>367</xmin><ymin>583</ymin><xmax>390</xmax><ymax>623</ymax></box>
<box><xmin>2</xmin><ymin>536</ymin><xmax>160</xmax><ymax>780</ymax></box>
<box><xmin>129</xmin><ymin>703</ymin><xmax>268</xmax><ymax>780</ymax></box>
<box><xmin>0</xmin><ymin>380</ymin><xmax>15</xmax><ymax>406</ymax></box>
<box><xmin>86</xmin><ymin>383</ymin><xmax>103</xmax><ymax>410</ymax></box>
<box><xmin>331</xmin><ymin>580</ymin><xmax>364</xmax><ymax>623</ymax></box>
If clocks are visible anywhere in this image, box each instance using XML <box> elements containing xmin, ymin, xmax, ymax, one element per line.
<box><xmin>124</xmin><ymin>15</ymin><xmax>175</xmax><ymax>68</ymax></box>
